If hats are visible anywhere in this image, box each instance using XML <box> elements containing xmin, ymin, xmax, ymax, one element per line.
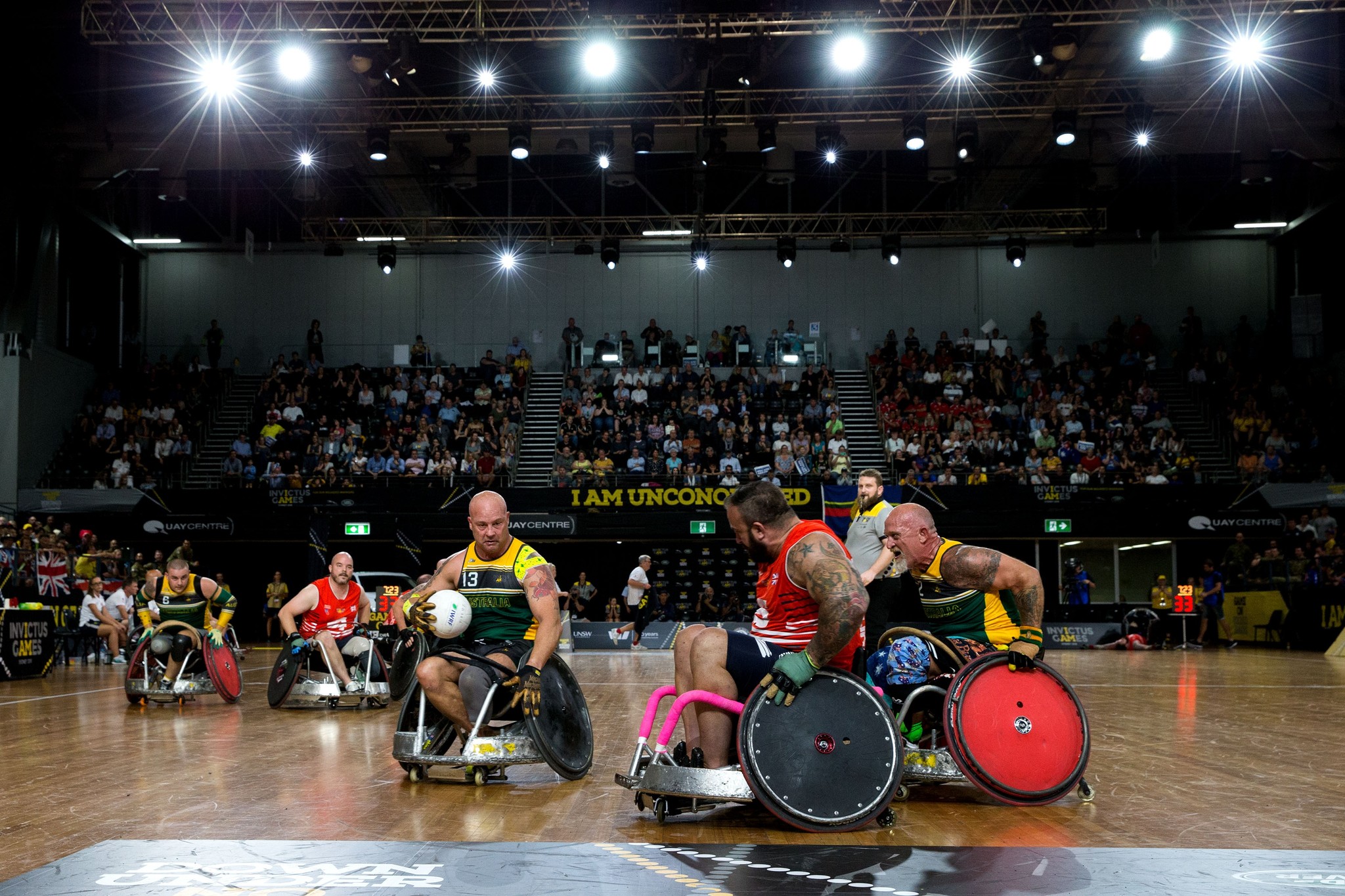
<box><xmin>571</xmin><ymin>585</ymin><xmax>579</xmax><ymax>590</ymax></box>
<box><xmin>23</xmin><ymin>524</ymin><xmax>32</xmax><ymax>529</ymax></box>
<box><xmin>835</xmin><ymin>430</ymin><xmax>843</xmax><ymax>435</ymax></box>
<box><xmin>1158</xmin><ymin>575</ymin><xmax>1166</xmax><ymax>579</ymax></box>
<box><xmin>928</xmin><ymin>433</ymin><xmax>935</xmax><ymax>439</ymax></box>
<box><xmin>913</xmin><ymin>434</ymin><xmax>920</xmax><ymax>438</ymax></box>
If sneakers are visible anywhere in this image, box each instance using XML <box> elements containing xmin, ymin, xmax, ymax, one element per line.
<box><xmin>631</xmin><ymin>643</ymin><xmax>647</xmax><ymax>650</ymax></box>
<box><xmin>611</xmin><ymin>628</ymin><xmax>620</xmax><ymax>645</ymax></box>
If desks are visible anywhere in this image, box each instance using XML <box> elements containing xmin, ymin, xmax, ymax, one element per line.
<box><xmin>1169</xmin><ymin>614</ymin><xmax>1203</xmax><ymax>650</ymax></box>
<box><xmin>0</xmin><ymin>607</ymin><xmax>54</xmax><ymax>682</ymax></box>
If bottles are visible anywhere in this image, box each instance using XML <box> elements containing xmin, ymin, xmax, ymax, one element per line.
<box><xmin>100</xmin><ymin>648</ymin><xmax>104</xmax><ymax>667</ymax></box>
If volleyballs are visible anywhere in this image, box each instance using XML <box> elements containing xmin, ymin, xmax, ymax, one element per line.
<box><xmin>423</xmin><ymin>589</ymin><xmax>472</xmax><ymax>639</ymax></box>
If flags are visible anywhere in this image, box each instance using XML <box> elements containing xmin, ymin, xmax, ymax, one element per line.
<box><xmin>37</xmin><ymin>550</ymin><xmax>71</xmax><ymax>597</ymax></box>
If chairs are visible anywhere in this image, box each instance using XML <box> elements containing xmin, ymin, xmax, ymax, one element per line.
<box><xmin>52</xmin><ymin>599</ymin><xmax>111</xmax><ymax>665</ymax></box>
<box><xmin>1253</xmin><ymin>610</ymin><xmax>1282</xmax><ymax>643</ymax></box>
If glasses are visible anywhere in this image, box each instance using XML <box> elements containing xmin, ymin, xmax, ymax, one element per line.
<box><xmin>92</xmin><ymin>582</ymin><xmax>103</xmax><ymax>586</ymax></box>
<box><xmin>896</xmin><ymin>442</ymin><xmax>898</xmax><ymax>446</ymax></box>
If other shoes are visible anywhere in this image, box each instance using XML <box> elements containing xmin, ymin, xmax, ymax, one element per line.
<box><xmin>159</xmin><ymin>676</ymin><xmax>174</xmax><ymax>690</ymax></box>
<box><xmin>466</xmin><ymin>734</ymin><xmax>502</xmax><ymax>775</ymax></box>
<box><xmin>896</xmin><ymin>713</ymin><xmax>923</xmax><ymax>749</ymax></box>
<box><xmin>345</xmin><ymin>680</ymin><xmax>361</xmax><ymax>696</ymax></box>
<box><xmin>1187</xmin><ymin>640</ymin><xmax>1203</xmax><ymax>648</ymax></box>
<box><xmin>647</xmin><ymin>741</ymin><xmax>731</xmax><ymax>805</ymax></box>
<box><xmin>1224</xmin><ymin>640</ymin><xmax>1237</xmax><ymax>649</ymax></box>
<box><xmin>111</xmin><ymin>656</ymin><xmax>127</xmax><ymax>665</ymax></box>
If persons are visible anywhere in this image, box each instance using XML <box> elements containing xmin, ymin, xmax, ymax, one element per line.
<box><xmin>278</xmin><ymin>551</ymin><xmax>381</xmax><ymax>693</ymax></box>
<box><xmin>866</xmin><ymin>503</ymin><xmax>1045</xmax><ymax>742</ymax></box>
<box><xmin>78</xmin><ymin>575</ymin><xmax>128</xmax><ymax>665</ymax></box>
<box><xmin>383</xmin><ymin>558</ymin><xmax>447</xmax><ymax>649</ymax></box>
<box><xmin>843</xmin><ymin>469</ymin><xmax>894</xmax><ymax>587</ymax></box>
<box><xmin>673</xmin><ymin>480</ymin><xmax>870</xmax><ymax>769</ymax></box>
<box><xmin>611</xmin><ymin>555</ymin><xmax>655</xmax><ymax>650</ymax></box>
<box><xmin>402</xmin><ymin>491</ymin><xmax>563</xmax><ymax>781</ymax></box>
<box><xmin>1187</xmin><ymin>558</ymin><xmax>1239</xmax><ymax>650</ymax></box>
<box><xmin>0</xmin><ymin>306</ymin><xmax>1345</xmax><ymax>651</ymax></box>
<box><xmin>135</xmin><ymin>559</ymin><xmax>237</xmax><ymax>690</ymax></box>
<box><xmin>1088</xmin><ymin>634</ymin><xmax>1161</xmax><ymax>650</ymax></box>
<box><xmin>266</xmin><ymin>571</ymin><xmax>288</xmax><ymax>643</ymax></box>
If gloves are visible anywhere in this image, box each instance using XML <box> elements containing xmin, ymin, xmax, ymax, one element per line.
<box><xmin>355</xmin><ymin>623</ymin><xmax>371</xmax><ymax>638</ymax></box>
<box><xmin>1007</xmin><ymin>626</ymin><xmax>1043</xmax><ymax>671</ymax></box>
<box><xmin>404</xmin><ymin>590</ymin><xmax>437</xmax><ymax>633</ymax></box>
<box><xmin>289</xmin><ymin>632</ymin><xmax>310</xmax><ymax>663</ymax></box>
<box><xmin>760</xmin><ymin>650</ymin><xmax>819</xmax><ymax>707</ymax></box>
<box><xmin>137</xmin><ymin>627</ymin><xmax>154</xmax><ymax>644</ymax></box>
<box><xmin>503</xmin><ymin>665</ymin><xmax>541</xmax><ymax>715</ymax></box>
<box><xmin>207</xmin><ymin>628</ymin><xmax>223</xmax><ymax>649</ymax></box>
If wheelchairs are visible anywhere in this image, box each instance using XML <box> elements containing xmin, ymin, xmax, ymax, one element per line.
<box><xmin>267</xmin><ymin>621</ymin><xmax>391</xmax><ymax>710</ymax></box>
<box><xmin>893</xmin><ymin>645</ymin><xmax>1096</xmax><ymax>804</ymax></box>
<box><xmin>125</xmin><ymin>622</ymin><xmax>159</xmax><ymax>658</ymax></box>
<box><xmin>388</xmin><ymin>631</ymin><xmax>431</xmax><ymax>701</ymax></box>
<box><xmin>613</xmin><ymin>646</ymin><xmax>906</xmax><ymax>833</ymax></box>
<box><xmin>392</xmin><ymin>647</ymin><xmax>594</xmax><ymax>786</ymax></box>
<box><xmin>124</xmin><ymin>620</ymin><xmax>244</xmax><ymax>706</ymax></box>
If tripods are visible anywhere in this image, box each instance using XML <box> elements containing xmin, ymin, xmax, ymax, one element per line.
<box><xmin>1056</xmin><ymin>579</ymin><xmax>1091</xmax><ymax>623</ymax></box>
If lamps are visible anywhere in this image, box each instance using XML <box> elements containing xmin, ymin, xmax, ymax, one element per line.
<box><xmin>816</xmin><ymin>123</ymin><xmax>848</xmax><ymax>164</ymax></box>
<box><xmin>903</xmin><ymin>114</ymin><xmax>927</xmax><ymax>150</ymax></box>
<box><xmin>882</xmin><ymin>234</ymin><xmax>902</xmax><ymax>265</ymax></box>
<box><xmin>631</xmin><ymin>119</ymin><xmax>655</xmax><ymax>154</ymax></box>
<box><xmin>952</xmin><ymin>120</ymin><xmax>975</xmax><ymax>159</ymax></box>
<box><xmin>347</xmin><ymin>31</ymin><xmax>417</xmax><ymax>86</ymax></box>
<box><xmin>377</xmin><ymin>245</ymin><xmax>397</xmax><ymax>274</ymax></box>
<box><xmin>509</xmin><ymin>123</ymin><xmax>532</xmax><ymax>160</ymax></box>
<box><xmin>830</xmin><ymin>239</ymin><xmax>851</xmax><ymax>252</ymax></box>
<box><xmin>776</xmin><ymin>238</ymin><xmax>796</xmax><ymax>268</ymax></box>
<box><xmin>697</xmin><ymin>126</ymin><xmax>728</xmax><ymax>166</ymax></box>
<box><xmin>574</xmin><ymin>237</ymin><xmax>620</xmax><ymax>270</ymax></box>
<box><xmin>690</xmin><ymin>240</ymin><xmax>710</xmax><ymax>270</ymax></box>
<box><xmin>1052</xmin><ymin>109</ymin><xmax>1077</xmax><ymax>145</ymax></box>
<box><xmin>589</xmin><ymin>129</ymin><xmax>613</xmax><ymax>169</ymax></box>
<box><xmin>366</xmin><ymin>127</ymin><xmax>391</xmax><ymax>160</ymax></box>
<box><xmin>1006</xmin><ymin>237</ymin><xmax>1027</xmax><ymax>268</ymax></box>
<box><xmin>755</xmin><ymin>119</ymin><xmax>779</xmax><ymax>152</ymax></box>
<box><xmin>446</xmin><ymin>132</ymin><xmax>471</xmax><ymax>164</ymax></box>
